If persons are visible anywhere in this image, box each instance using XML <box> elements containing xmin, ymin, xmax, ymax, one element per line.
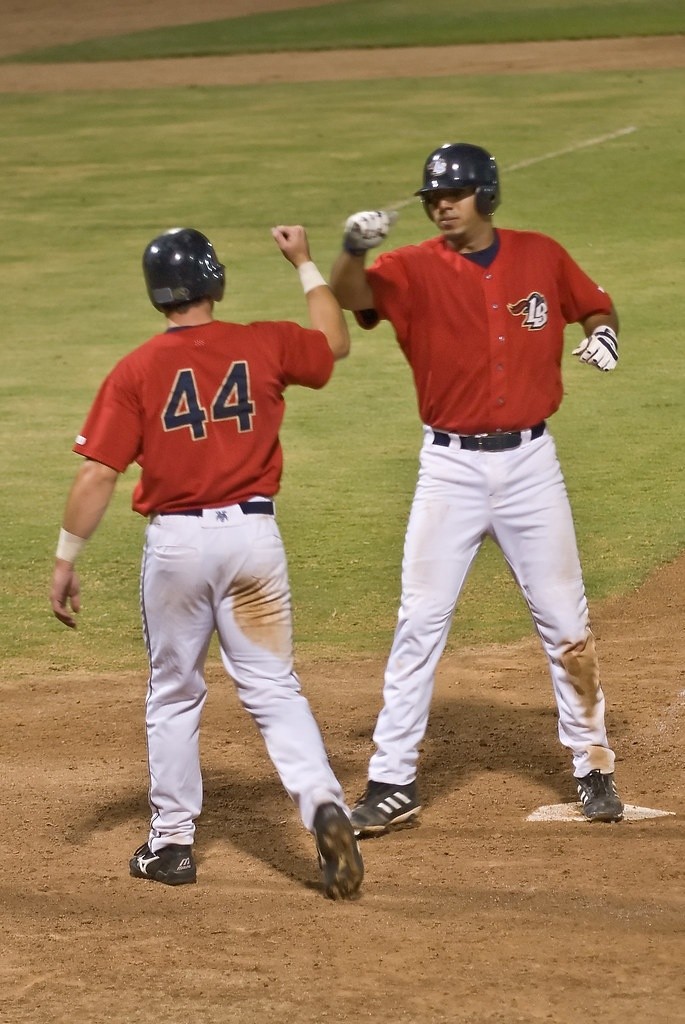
<box><xmin>50</xmin><ymin>225</ymin><xmax>368</xmax><ymax>901</ymax></box>
<box><xmin>332</xmin><ymin>142</ymin><xmax>624</xmax><ymax>830</ymax></box>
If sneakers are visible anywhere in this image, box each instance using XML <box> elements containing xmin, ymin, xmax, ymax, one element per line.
<box><xmin>577</xmin><ymin>768</ymin><xmax>626</xmax><ymax>821</ymax></box>
<box><xmin>348</xmin><ymin>780</ymin><xmax>421</xmax><ymax>835</ymax></box>
<box><xmin>314</xmin><ymin>803</ymin><xmax>364</xmax><ymax>898</ymax></box>
<box><xmin>129</xmin><ymin>841</ymin><xmax>198</xmax><ymax>886</ymax></box>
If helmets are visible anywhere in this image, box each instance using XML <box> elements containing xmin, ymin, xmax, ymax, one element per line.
<box><xmin>412</xmin><ymin>142</ymin><xmax>500</xmax><ymax>221</ymax></box>
<box><xmin>142</xmin><ymin>228</ymin><xmax>224</xmax><ymax>308</ymax></box>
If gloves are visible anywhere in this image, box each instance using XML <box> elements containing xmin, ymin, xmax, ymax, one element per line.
<box><xmin>342</xmin><ymin>208</ymin><xmax>397</xmax><ymax>253</ymax></box>
<box><xmin>571</xmin><ymin>325</ymin><xmax>620</xmax><ymax>371</ymax></box>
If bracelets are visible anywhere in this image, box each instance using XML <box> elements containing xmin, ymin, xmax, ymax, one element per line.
<box><xmin>55</xmin><ymin>526</ymin><xmax>86</xmax><ymax>564</ymax></box>
<box><xmin>298</xmin><ymin>259</ymin><xmax>328</xmax><ymax>296</ymax></box>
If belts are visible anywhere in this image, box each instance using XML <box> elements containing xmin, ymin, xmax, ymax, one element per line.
<box><xmin>434</xmin><ymin>423</ymin><xmax>547</xmax><ymax>451</ymax></box>
<box><xmin>161</xmin><ymin>502</ymin><xmax>274</xmax><ymax>516</ymax></box>
